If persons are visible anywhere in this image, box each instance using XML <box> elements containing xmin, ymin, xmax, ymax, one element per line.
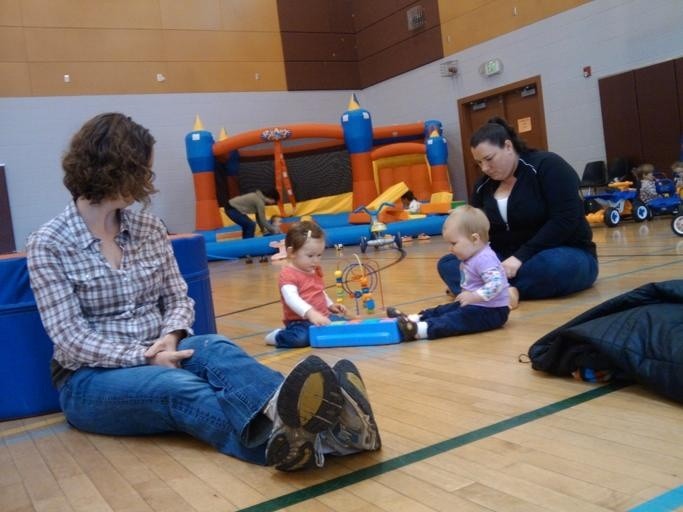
<box><xmin>387</xmin><ymin>204</ymin><xmax>510</xmax><ymax>341</ymax></box>
<box><xmin>402</xmin><ymin>191</ymin><xmax>420</xmax><ymax>209</ymax></box>
<box><xmin>264</xmin><ymin>221</ymin><xmax>347</xmax><ymax>348</ymax></box>
<box><xmin>224</xmin><ymin>185</ymin><xmax>283</xmax><ymax>238</ymax></box>
<box><xmin>437</xmin><ymin>116</ymin><xmax>598</xmax><ymax>299</ymax></box>
<box><xmin>27</xmin><ymin>112</ymin><xmax>381</xmax><ymax>472</ymax></box>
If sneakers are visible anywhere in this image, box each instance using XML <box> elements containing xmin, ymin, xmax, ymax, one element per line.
<box><xmin>265</xmin><ymin>355</ymin><xmax>381</xmax><ymax>473</ymax></box>
<box><xmin>388</xmin><ymin>307</ymin><xmax>417</xmax><ymax>341</ymax></box>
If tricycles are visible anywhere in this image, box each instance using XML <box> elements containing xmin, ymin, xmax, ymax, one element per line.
<box><xmin>353</xmin><ymin>201</ymin><xmax>402</xmax><ymax>253</ymax></box>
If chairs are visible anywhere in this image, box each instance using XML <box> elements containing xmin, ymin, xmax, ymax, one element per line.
<box><xmin>608</xmin><ymin>155</ymin><xmax>637</xmax><ymax>180</ymax></box>
<box><xmin>577</xmin><ymin>160</ymin><xmax>606</xmax><ymax>196</ymax></box>
